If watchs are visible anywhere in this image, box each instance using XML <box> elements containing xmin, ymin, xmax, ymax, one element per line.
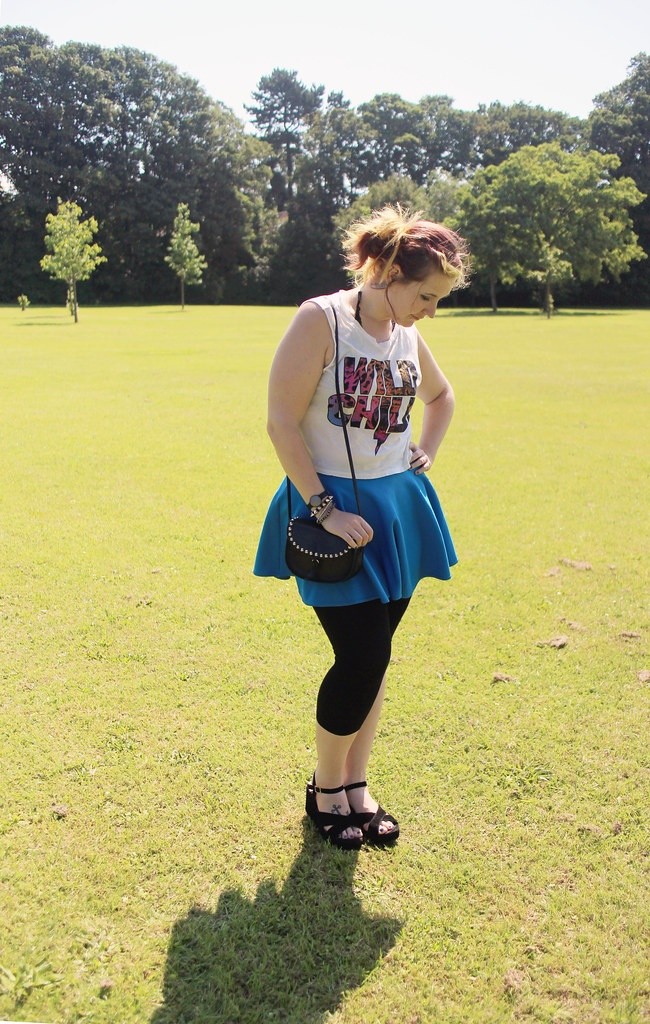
<box><xmin>307</xmin><ymin>490</ymin><xmax>329</xmax><ymax>509</ymax></box>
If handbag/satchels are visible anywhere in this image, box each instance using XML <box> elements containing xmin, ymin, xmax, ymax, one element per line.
<box><xmin>285</xmin><ymin>517</ymin><xmax>362</xmax><ymax>583</ymax></box>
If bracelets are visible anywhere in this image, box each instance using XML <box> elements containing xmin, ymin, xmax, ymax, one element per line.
<box><xmin>310</xmin><ymin>495</ymin><xmax>336</xmax><ymax>524</ymax></box>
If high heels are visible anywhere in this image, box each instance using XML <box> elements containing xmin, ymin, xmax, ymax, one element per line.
<box><xmin>344</xmin><ymin>780</ymin><xmax>399</xmax><ymax>840</ymax></box>
<box><xmin>305</xmin><ymin>772</ymin><xmax>363</xmax><ymax>848</ymax></box>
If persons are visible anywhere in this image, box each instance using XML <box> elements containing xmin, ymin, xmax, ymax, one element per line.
<box><xmin>251</xmin><ymin>210</ymin><xmax>466</xmax><ymax>849</ymax></box>
